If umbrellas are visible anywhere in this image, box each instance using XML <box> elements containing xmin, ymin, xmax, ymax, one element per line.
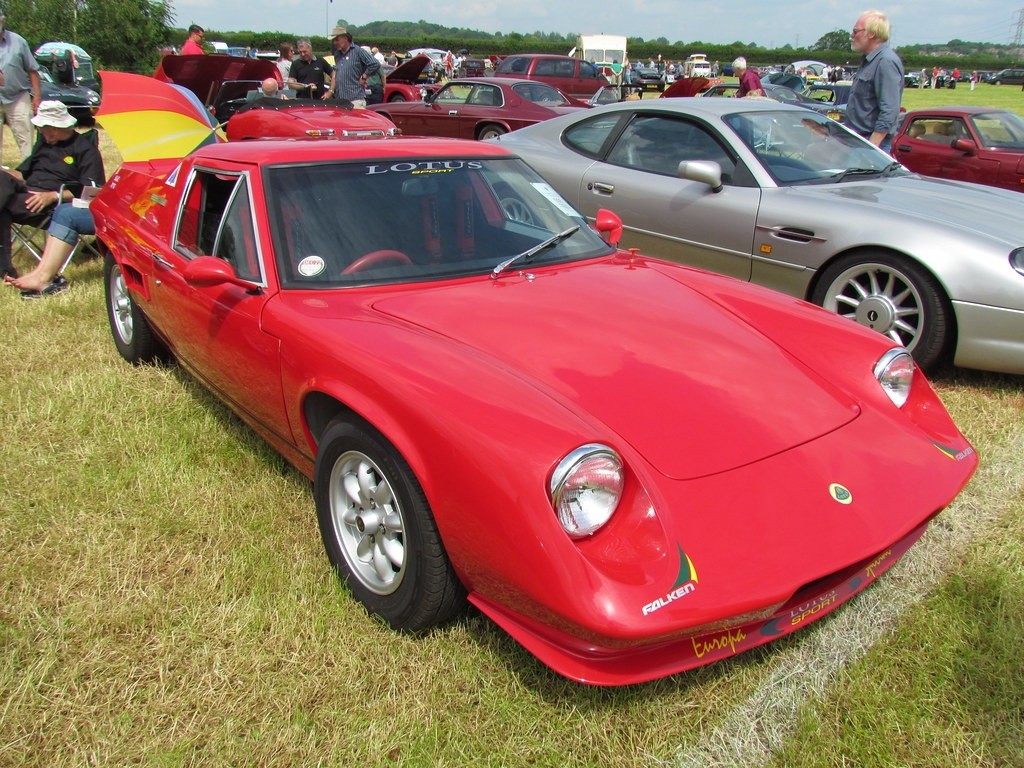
<box><xmin>91</xmin><ymin>69</ymin><xmax>229</xmax><ymax>161</ymax></box>
<box><xmin>34</xmin><ymin>40</ymin><xmax>92</xmax><ymax>62</ymax></box>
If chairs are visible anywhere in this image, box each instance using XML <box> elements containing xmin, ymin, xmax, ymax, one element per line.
<box><xmin>8</xmin><ymin>128</ymin><xmax>107</xmax><ymax>276</ymax></box>
<box><xmin>909</xmin><ymin>122</ymin><xmax>958</xmax><ymax>144</ymax></box>
<box><xmin>215</xmin><ymin>173</ymin><xmax>339</xmax><ymax>282</ymax></box>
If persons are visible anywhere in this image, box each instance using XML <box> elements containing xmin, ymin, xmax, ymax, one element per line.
<box><xmin>971</xmin><ymin>71</ymin><xmax>977</xmax><ymax>90</ymax></box>
<box><xmin>260</xmin><ymin>27</ymin><xmax>381</xmax><ymax>109</ymax></box>
<box><xmin>372</xmin><ymin>47</ymin><xmax>398</xmax><ymax>67</ymax></box>
<box><xmin>732</xmin><ymin>58</ymin><xmax>773</xmax><ymax>150</ymax></box>
<box><xmin>484</xmin><ymin>55</ymin><xmax>501</xmax><ymax>70</ymax></box>
<box><xmin>441</xmin><ymin>51</ymin><xmax>472</xmax><ymax>80</ymax></box>
<box><xmin>713</xmin><ymin>61</ymin><xmax>719</xmax><ymax>77</ymax></box>
<box><xmin>785</xmin><ymin>63</ymin><xmax>807</xmax><ymax>83</ymax></box>
<box><xmin>0</xmin><ymin>9</ymin><xmax>41</xmax><ymax>167</ymax></box>
<box><xmin>625</xmin><ymin>57</ymin><xmax>685</xmax><ymax>95</ymax></box>
<box><xmin>799</xmin><ymin>117</ymin><xmax>873</xmax><ymax>150</ymax></box>
<box><xmin>0</xmin><ymin>100</ymin><xmax>100</xmax><ymax>277</ymax></box>
<box><xmin>609</xmin><ymin>60</ymin><xmax>623</xmax><ymax>88</ymax></box>
<box><xmin>822</xmin><ymin>65</ymin><xmax>843</xmax><ymax>83</ymax></box>
<box><xmin>182</xmin><ymin>25</ymin><xmax>205</xmax><ymax>54</ymax></box>
<box><xmin>3</xmin><ymin>202</ymin><xmax>96</xmax><ymax>291</ymax></box>
<box><xmin>919</xmin><ymin>66</ymin><xmax>959</xmax><ymax>89</ymax></box>
<box><xmin>844</xmin><ymin>9</ymin><xmax>904</xmax><ymax>152</ymax></box>
<box><xmin>591</xmin><ymin>59</ymin><xmax>598</xmax><ymax>69</ymax></box>
<box><xmin>51</xmin><ymin>49</ymin><xmax>80</xmax><ymax>86</ymax></box>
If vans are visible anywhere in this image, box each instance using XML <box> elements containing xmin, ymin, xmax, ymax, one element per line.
<box><xmin>685</xmin><ymin>61</ymin><xmax>712</xmax><ymax>79</ymax></box>
<box><xmin>490</xmin><ymin>55</ymin><xmax>634</xmax><ymax>107</ymax></box>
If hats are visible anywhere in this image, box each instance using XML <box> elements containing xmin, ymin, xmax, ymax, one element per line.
<box><xmin>327</xmin><ymin>26</ymin><xmax>346</xmax><ymax>41</ymax></box>
<box><xmin>31</xmin><ymin>100</ymin><xmax>78</xmax><ymax>128</ymax></box>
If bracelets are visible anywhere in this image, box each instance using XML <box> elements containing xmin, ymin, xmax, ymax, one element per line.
<box><xmin>56</xmin><ymin>190</ymin><xmax>61</xmax><ymax>198</ymax></box>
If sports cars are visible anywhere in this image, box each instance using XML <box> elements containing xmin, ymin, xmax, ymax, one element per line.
<box><xmin>478</xmin><ymin>98</ymin><xmax>1024</xmax><ymax>378</ymax></box>
<box><xmin>153</xmin><ymin>48</ymin><xmax>402</xmax><ymax>145</ymax></box>
<box><xmin>89</xmin><ymin>136</ymin><xmax>983</xmax><ymax>687</ymax></box>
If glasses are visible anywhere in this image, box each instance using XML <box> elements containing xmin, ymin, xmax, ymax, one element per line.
<box><xmin>853</xmin><ymin>28</ymin><xmax>864</xmax><ymax>34</ymax></box>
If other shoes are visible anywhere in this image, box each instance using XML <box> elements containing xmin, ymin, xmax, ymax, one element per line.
<box><xmin>22</xmin><ymin>276</ymin><xmax>68</xmax><ymax>301</ymax></box>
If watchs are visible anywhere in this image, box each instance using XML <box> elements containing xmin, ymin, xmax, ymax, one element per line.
<box><xmin>361</xmin><ymin>74</ymin><xmax>366</xmax><ymax>80</ymax></box>
<box><xmin>329</xmin><ymin>89</ymin><xmax>334</xmax><ymax>93</ymax></box>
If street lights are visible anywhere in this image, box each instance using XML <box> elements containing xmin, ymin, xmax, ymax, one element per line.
<box><xmin>325</xmin><ymin>0</ymin><xmax>333</xmax><ymax>37</ymax></box>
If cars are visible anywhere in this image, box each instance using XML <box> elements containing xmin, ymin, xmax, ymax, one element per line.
<box><xmin>629</xmin><ymin>68</ymin><xmax>666</xmax><ymax>92</ymax></box>
<box><xmin>632</xmin><ymin>54</ymin><xmax>995</xmax><ymax>157</ymax></box>
<box><xmin>28</xmin><ymin>62</ymin><xmax>102</xmax><ymax>122</ymax></box>
<box><xmin>377</xmin><ymin>52</ymin><xmax>454</xmax><ymax>105</ymax></box>
<box><xmin>988</xmin><ymin>68</ymin><xmax>1024</xmax><ymax>85</ymax></box>
<box><xmin>360</xmin><ymin>75</ymin><xmax>630</xmax><ymax>143</ymax></box>
<box><xmin>890</xmin><ymin>104</ymin><xmax>1024</xmax><ymax>191</ymax></box>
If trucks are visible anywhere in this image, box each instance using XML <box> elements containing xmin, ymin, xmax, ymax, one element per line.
<box><xmin>208</xmin><ymin>42</ymin><xmax>250</xmax><ymax>56</ymax></box>
<box><xmin>574</xmin><ymin>35</ymin><xmax>628</xmax><ymax>83</ymax></box>
